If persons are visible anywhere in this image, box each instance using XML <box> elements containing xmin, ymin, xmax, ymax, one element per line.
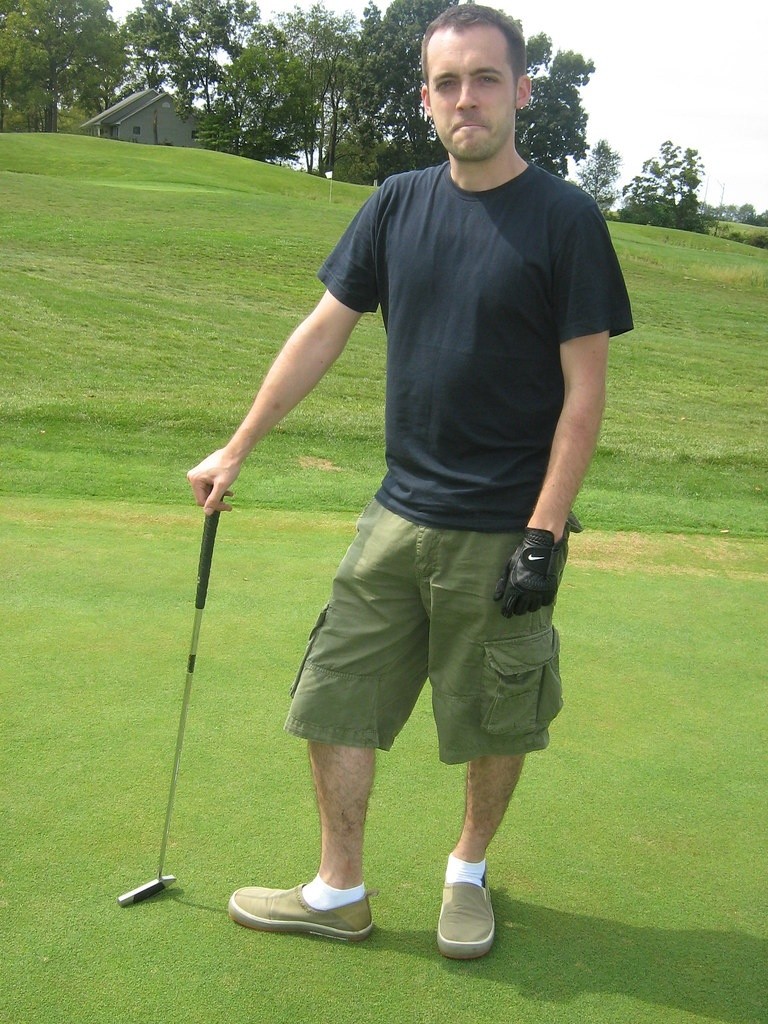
<box><xmin>185</xmin><ymin>4</ymin><xmax>634</xmax><ymax>963</ymax></box>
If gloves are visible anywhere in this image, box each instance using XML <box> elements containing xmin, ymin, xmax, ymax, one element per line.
<box><xmin>493</xmin><ymin>528</ymin><xmax>561</xmax><ymax>619</ymax></box>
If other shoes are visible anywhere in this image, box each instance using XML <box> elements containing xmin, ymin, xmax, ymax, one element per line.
<box><xmin>229</xmin><ymin>882</ymin><xmax>380</xmax><ymax>942</ymax></box>
<box><xmin>437</xmin><ymin>859</ymin><xmax>494</xmax><ymax>958</ymax></box>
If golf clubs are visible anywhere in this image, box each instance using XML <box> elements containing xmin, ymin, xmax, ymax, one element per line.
<box><xmin>115</xmin><ymin>484</ymin><xmax>227</xmax><ymax>909</ymax></box>
